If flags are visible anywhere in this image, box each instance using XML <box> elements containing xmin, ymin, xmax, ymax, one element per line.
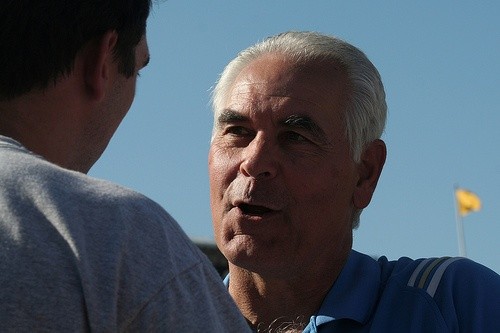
<box><xmin>457</xmin><ymin>187</ymin><xmax>481</xmax><ymax>216</ymax></box>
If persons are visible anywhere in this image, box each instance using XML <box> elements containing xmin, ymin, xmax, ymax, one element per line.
<box><xmin>209</xmin><ymin>31</ymin><xmax>500</xmax><ymax>333</ymax></box>
<box><xmin>0</xmin><ymin>0</ymin><xmax>253</xmax><ymax>333</ymax></box>
